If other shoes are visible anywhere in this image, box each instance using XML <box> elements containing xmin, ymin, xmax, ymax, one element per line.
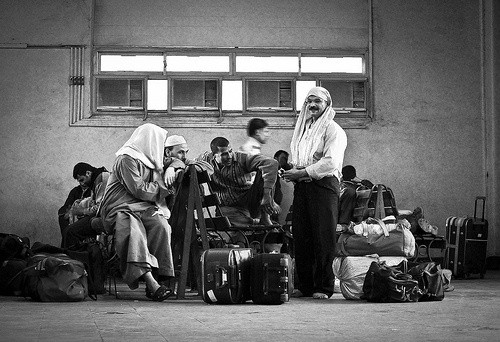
<box><xmin>290</xmin><ymin>289</ymin><xmax>304</xmax><ymax>297</ymax></box>
<box><xmin>417</xmin><ymin>218</ymin><xmax>439</xmax><ymax>236</ymax></box>
<box><xmin>411</xmin><ymin>207</ymin><xmax>424</xmax><ymax>219</ymax></box>
<box><xmin>314</xmin><ymin>292</ymin><xmax>329</xmax><ymax>299</ymax></box>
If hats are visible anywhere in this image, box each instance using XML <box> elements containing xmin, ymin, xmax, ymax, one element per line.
<box><xmin>164</xmin><ymin>135</ymin><xmax>186</xmax><ymax>147</ymax></box>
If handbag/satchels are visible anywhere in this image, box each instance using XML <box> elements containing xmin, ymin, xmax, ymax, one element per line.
<box><xmin>366</xmin><ymin>184</ymin><xmax>400</xmax><ymax>216</ymax></box>
<box><xmin>333</xmin><ymin>217</ymin><xmax>415</xmax><ymax>258</ymax></box>
<box><xmin>404</xmin><ymin>260</ymin><xmax>455</xmax><ymax>300</ymax></box>
<box><xmin>363</xmin><ymin>261</ymin><xmax>418</xmax><ymax>304</ymax></box>
<box><xmin>0</xmin><ymin>233</ymin><xmax>97</xmax><ymax>302</ymax></box>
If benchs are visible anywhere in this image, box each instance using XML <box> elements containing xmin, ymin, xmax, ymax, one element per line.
<box><xmin>82</xmin><ymin>163</ymin><xmax>448</xmax><ymax>301</ymax></box>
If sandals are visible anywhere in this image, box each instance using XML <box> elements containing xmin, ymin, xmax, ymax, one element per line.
<box><xmin>145</xmin><ymin>285</ymin><xmax>172</xmax><ymax>301</ymax></box>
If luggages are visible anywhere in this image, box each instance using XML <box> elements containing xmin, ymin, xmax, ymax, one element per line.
<box><xmin>249</xmin><ymin>254</ymin><xmax>294</xmax><ymax>305</ymax></box>
<box><xmin>445</xmin><ymin>195</ymin><xmax>488</xmax><ymax>278</ymax></box>
<box><xmin>200</xmin><ymin>247</ymin><xmax>252</xmax><ymax>304</ymax></box>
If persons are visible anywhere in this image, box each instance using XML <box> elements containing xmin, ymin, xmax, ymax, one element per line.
<box><xmin>163</xmin><ymin>134</ymin><xmax>214</xmax><ymax>210</ymax></box>
<box><xmin>273</xmin><ymin>150</ymin><xmax>293</xmax><ymax>171</ymax></box>
<box><xmin>278</xmin><ymin>86</ymin><xmax>348</xmax><ymax>298</ymax></box>
<box><xmin>100</xmin><ymin>122</ymin><xmax>184</xmax><ymax>302</ymax></box>
<box><xmin>63</xmin><ymin>161</ymin><xmax>113</xmax><ymax>302</ymax></box>
<box><xmin>58</xmin><ymin>182</ymin><xmax>91</xmax><ymax>249</ymax></box>
<box><xmin>193</xmin><ymin>137</ymin><xmax>278</xmax><ymax>234</ymax></box>
<box><xmin>239</xmin><ymin>118</ymin><xmax>270</xmax><ymax>155</ymax></box>
